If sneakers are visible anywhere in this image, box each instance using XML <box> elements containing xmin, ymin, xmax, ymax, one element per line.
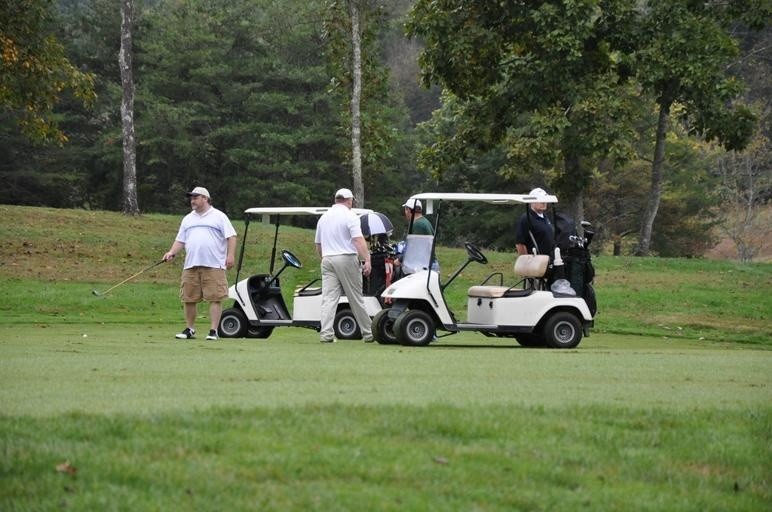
<box><xmin>175</xmin><ymin>327</ymin><xmax>197</xmax><ymax>340</ymax></box>
<box><xmin>205</xmin><ymin>328</ymin><xmax>219</xmax><ymax>341</ymax></box>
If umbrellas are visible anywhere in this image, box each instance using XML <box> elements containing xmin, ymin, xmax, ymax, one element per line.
<box><xmin>358</xmin><ymin>212</ymin><xmax>395</xmax><ymax>239</ymax></box>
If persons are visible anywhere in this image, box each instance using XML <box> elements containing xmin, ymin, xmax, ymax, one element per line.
<box><xmin>394</xmin><ymin>199</ymin><xmax>440</xmax><ymax>276</ymax></box>
<box><xmin>314</xmin><ymin>189</ymin><xmax>375</xmax><ymax>343</ymax></box>
<box><xmin>163</xmin><ymin>188</ymin><xmax>237</xmax><ymax>340</ymax></box>
<box><xmin>515</xmin><ymin>188</ymin><xmax>557</xmax><ymax>291</ymax></box>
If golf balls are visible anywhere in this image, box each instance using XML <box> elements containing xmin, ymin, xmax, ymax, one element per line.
<box><xmin>83</xmin><ymin>334</ymin><xmax>88</xmax><ymax>338</ymax></box>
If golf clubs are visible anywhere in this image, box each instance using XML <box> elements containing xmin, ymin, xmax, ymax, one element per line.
<box><xmin>582</xmin><ymin>220</ymin><xmax>591</xmax><ymax>236</ymax></box>
<box><xmin>92</xmin><ymin>254</ymin><xmax>177</xmax><ymax>297</ymax></box>
<box><xmin>569</xmin><ymin>235</ymin><xmax>587</xmax><ymax>247</ymax></box>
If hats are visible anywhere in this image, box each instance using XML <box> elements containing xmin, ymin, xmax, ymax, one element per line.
<box><xmin>529</xmin><ymin>189</ymin><xmax>552</xmax><ymax>201</ymax></box>
<box><xmin>186</xmin><ymin>186</ymin><xmax>211</xmax><ymax>199</ymax></box>
<box><xmin>335</xmin><ymin>187</ymin><xmax>356</xmax><ymax>198</ymax></box>
<box><xmin>401</xmin><ymin>197</ymin><xmax>426</xmax><ymax>210</ymax></box>
